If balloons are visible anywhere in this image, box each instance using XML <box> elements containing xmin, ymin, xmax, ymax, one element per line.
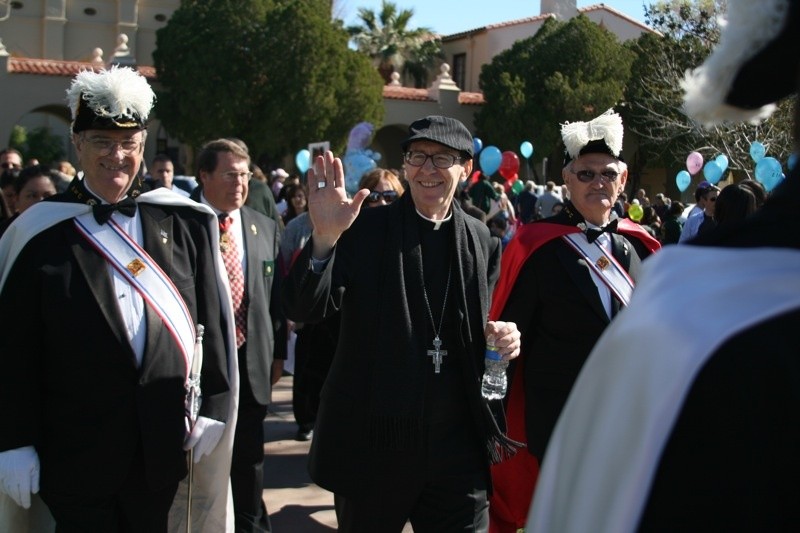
<box><xmin>295</xmin><ymin>122</ymin><xmax>796</xmax><ymax>220</ymax></box>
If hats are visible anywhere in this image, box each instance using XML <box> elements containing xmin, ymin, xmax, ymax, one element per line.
<box><xmin>276</xmin><ymin>169</ymin><xmax>288</xmax><ymax>178</ymax></box>
<box><xmin>682</xmin><ymin>0</ymin><xmax>800</xmax><ymax>129</ymax></box>
<box><xmin>561</xmin><ymin>107</ymin><xmax>632</xmax><ymax>168</ymax></box>
<box><xmin>66</xmin><ymin>60</ymin><xmax>158</xmax><ymax>130</ymax></box>
<box><xmin>404</xmin><ymin>115</ymin><xmax>474</xmax><ymax>160</ymax></box>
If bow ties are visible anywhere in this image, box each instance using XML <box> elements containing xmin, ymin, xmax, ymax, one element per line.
<box><xmin>587</xmin><ymin>219</ymin><xmax>618</xmax><ymax>244</ymax></box>
<box><xmin>92</xmin><ymin>196</ymin><xmax>137</xmax><ymax>226</ymax></box>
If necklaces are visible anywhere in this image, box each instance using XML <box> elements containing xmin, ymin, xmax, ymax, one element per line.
<box><xmin>424</xmin><ymin>267</ymin><xmax>451</xmax><ymax>373</ymax></box>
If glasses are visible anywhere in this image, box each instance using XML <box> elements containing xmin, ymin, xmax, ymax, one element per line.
<box><xmin>703</xmin><ymin>196</ymin><xmax>719</xmax><ymax>202</ymax></box>
<box><xmin>365</xmin><ymin>191</ymin><xmax>398</xmax><ymax>203</ymax></box>
<box><xmin>209</xmin><ymin>172</ymin><xmax>252</xmax><ymax>184</ymax></box>
<box><xmin>404</xmin><ymin>150</ymin><xmax>461</xmax><ymax>170</ymax></box>
<box><xmin>76</xmin><ymin>133</ymin><xmax>145</xmax><ymax>159</ymax></box>
<box><xmin>570</xmin><ymin>168</ymin><xmax>624</xmax><ymax>183</ymax></box>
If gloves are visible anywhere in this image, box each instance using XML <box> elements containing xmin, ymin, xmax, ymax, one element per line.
<box><xmin>0</xmin><ymin>446</ymin><xmax>40</xmax><ymax>509</ymax></box>
<box><xmin>184</xmin><ymin>415</ymin><xmax>226</xmax><ymax>463</ymax></box>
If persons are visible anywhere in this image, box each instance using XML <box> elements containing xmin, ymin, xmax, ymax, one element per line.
<box><xmin>270</xmin><ymin>116</ymin><xmax>520</xmax><ymax>533</ymax></box>
<box><xmin>185</xmin><ymin>138</ymin><xmax>276</xmax><ymax>533</ymax></box>
<box><xmin>0</xmin><ymin>150</ymin><xmax>768</xmax><ymax>471</ymax></box>
<box><xmin>0</xmin><ymin>68</ymin><xmax>238</xmax><ymax>533</ymax></box>
<box><xmin>524</xmin><ymin>0</ymin><xmax>800</xmax><ymax>533</ymax></box>
<box><xmin>492</xmin><ymin>108</ymin><xmax>663</xmax><ymax>533</ymax></box>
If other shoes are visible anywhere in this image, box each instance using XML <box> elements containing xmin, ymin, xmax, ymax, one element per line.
<box><xmin>295</xmin><ymin>428</ymin><xmax>314</xmax><ymax>441</ymax></box>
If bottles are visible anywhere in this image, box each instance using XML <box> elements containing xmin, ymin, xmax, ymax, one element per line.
<box><xmin>481</xmin><ymin>332</ymin><xmax>509</xmax><ymax>400</ymax></box>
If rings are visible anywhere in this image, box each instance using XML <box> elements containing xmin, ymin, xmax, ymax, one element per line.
<box><xmin>317</xmin><ymin>181</ymin><xmax>326</xmax><ymax>188</ymax></box>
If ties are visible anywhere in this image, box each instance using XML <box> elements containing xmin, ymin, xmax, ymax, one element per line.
<box><xmin>217</xmin><ymin>212</ymin><xmax>247</xmax><ymax>350</ymax></box>
